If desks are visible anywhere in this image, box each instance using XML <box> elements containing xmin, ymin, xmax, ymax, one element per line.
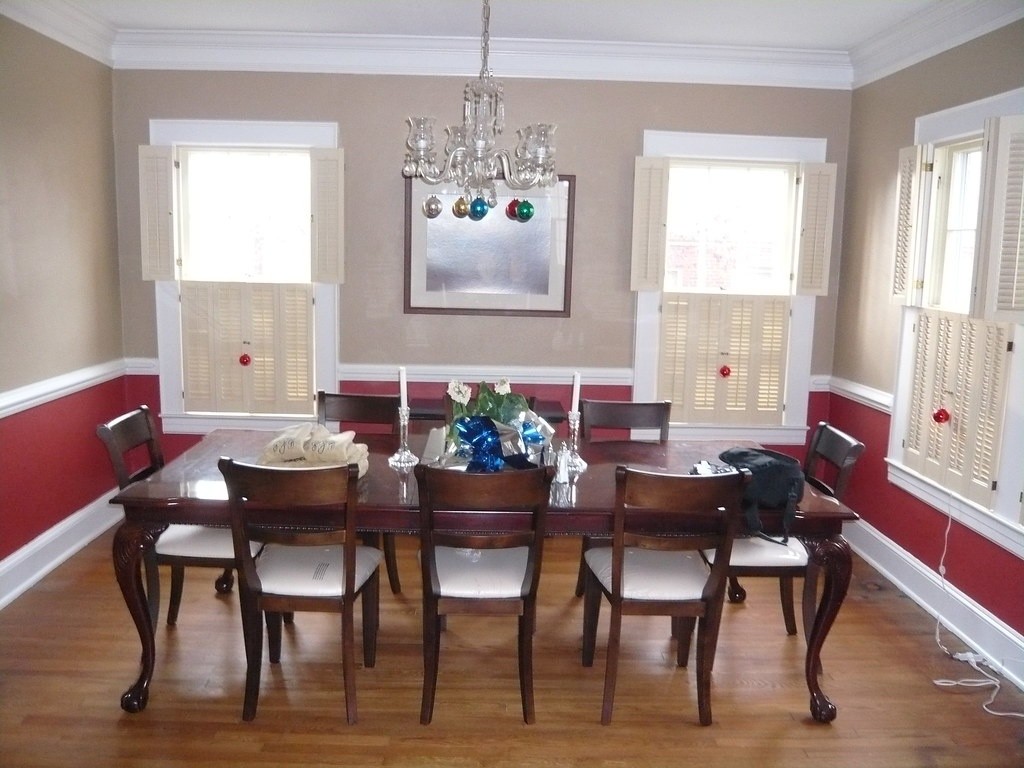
<box><xmin>107</xmin><ymin>424</ymin><xmax>861</xmax><ymax>725</ymax></box>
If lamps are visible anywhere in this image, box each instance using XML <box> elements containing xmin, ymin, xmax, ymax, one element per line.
<box><xmin>401</xmin><ymin>1</ymin><xmax>560</xmax><ymax>223</ymax></box>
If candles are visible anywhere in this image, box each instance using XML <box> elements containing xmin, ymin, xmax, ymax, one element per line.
<box><xmin>571</xmin><ymin>371</ymin><xmax>580</xmax><ymax>412</ymax></box>
<box><xmin>399</xmin><ymin>364</ymin><xmax>407</xmax><ymax>407</ymax></box>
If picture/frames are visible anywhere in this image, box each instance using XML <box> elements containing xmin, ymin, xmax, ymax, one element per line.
<box><xmin>403</xmin><ymin>168</ymin><xmax>576</xmax><ymax>319</ymax></box>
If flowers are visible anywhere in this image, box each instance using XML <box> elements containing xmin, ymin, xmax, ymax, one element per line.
<box><xmin>446</xmin><ymin>375</ymin><xmax>529</xmax><ymax>452</ymax></box>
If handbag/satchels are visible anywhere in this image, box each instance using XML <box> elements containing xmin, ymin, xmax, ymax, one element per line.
<box><xmin>719</xmin><ymin>447</ymin><xmax>805</xmax><ymax>547</ymax></box>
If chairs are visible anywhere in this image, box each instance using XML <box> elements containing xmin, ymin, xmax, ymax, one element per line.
<box><xmin>411</xmin><ymin>461</ymin><xmax>555</xmax><ymax>727</ymax></box>
<box><xmin>96</xmin><ymin>405</ymin><xmax>266</xmax><ymax>637</ymax></box>
<box><xmin>694</xmin><ymin>420</ymin><xmax>868</xmax><ymax>676</ymax></box>
<box><xmin>218</xmin><ymin>454</ymin><xmax>386</xmax><ymax>728</ymax></box>
<box><xmin>582</xmin><ymin>463</ymin><xmax>754</xmax><ymax>724</ymax></box>
<box><xmin>307</xmin><ymin>391</ymin><xmax>670</xmax><ymax>609</ymax></box>
